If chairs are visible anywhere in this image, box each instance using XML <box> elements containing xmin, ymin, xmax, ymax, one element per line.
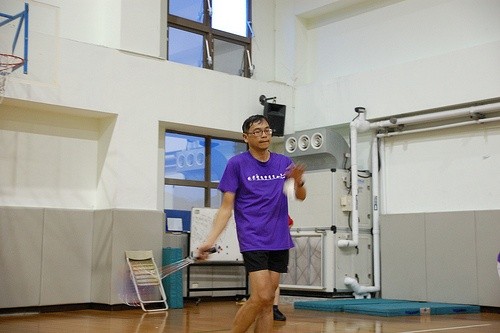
<box><xmin>125</xmin><ymin>250</ymin><xmax>169</xmax><ymax>312</ymax></box>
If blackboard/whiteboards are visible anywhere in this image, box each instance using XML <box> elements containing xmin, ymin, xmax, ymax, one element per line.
<box><xmin>187</xmin><ymin>207</ymin><xmax>245</xmax><ymax>266</ymax></box>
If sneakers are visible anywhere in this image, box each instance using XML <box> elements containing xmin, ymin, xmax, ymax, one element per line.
<box><xmin>273</xmin><ymin>309</ymin><xmax>287</xmax><ymax>321</ymax></box>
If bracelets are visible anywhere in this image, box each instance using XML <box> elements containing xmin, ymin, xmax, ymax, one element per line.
<box><xmin>295</xmin><ymin>183</ymin><xmax>305</xmax><ymax>188</ymax></box>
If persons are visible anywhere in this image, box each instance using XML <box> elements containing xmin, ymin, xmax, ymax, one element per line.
<box><xmin>198</xmin><ymin>115</ymin><xmax>306</xmax><ymax>333</ymax></box>
<box><xmin>272</xmin><ymin>214</ymin><xmax>294</xmax><ymax>322</ymax></box>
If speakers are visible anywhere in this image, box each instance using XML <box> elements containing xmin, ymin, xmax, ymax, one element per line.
<box><xmin>263</xmin><ymin>103</ymin><xmax>286</xmax><ymax>137</ymax></box>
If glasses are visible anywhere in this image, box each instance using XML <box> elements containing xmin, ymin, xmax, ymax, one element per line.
<box><xmin>246</xmin><ymin>129</ymin><xmax>273</xmax><ymax>136</ymax></box>
